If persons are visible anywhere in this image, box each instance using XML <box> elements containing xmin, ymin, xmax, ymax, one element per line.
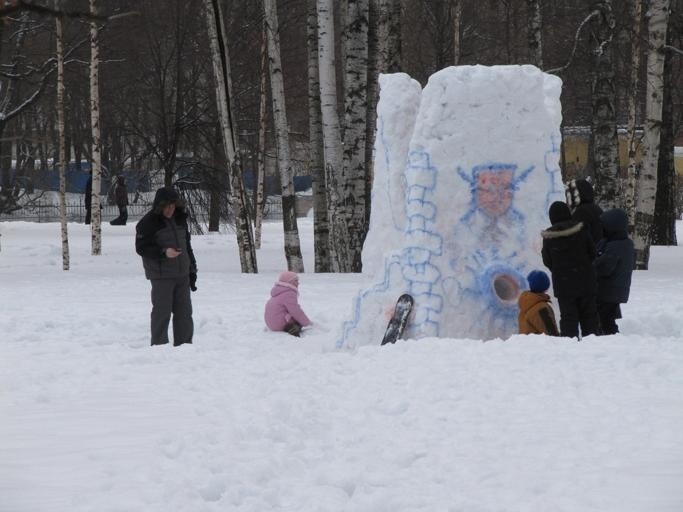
<box><xmin>264</xmin><ymin>271</ymin><xmax>313</xmax><ymax>337</ymax></box>
<box><xmin>111</xmin><ymin>175</ymin><xmax>129</xmax><ymax>225</ymax></box>
<box><xmin>136</xmin><ymin>187</ymin><xmax>197</xmax><ymax>346</ymax></box>
<box><xmin>518</xmin><ymin>270</ymin><xmax>559</xmax><ymax>336</ymax></box>
<box><xmin>542</xmin><ymin>179</ymin><xmax>634</xmax><ymax>341</ymax></box>
<box><xmin>85</xmin><ymin>170</ymin><xmax>103</xmax><ymax>224</ymax></box>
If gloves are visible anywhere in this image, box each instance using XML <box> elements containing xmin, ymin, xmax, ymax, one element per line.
<box><xmin>190</xmin><ymin>272</ymin><xmax>197</xmax><ymax>291</ymax></box>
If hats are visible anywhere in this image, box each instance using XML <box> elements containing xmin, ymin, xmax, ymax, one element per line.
<box><xmin>528</xmin><ymin>270</ymin><xmax>550</xmax><ymax>291</ymax></box>
<box><xmin>279</xmin><ymin>272</ymin><xmax>298</xmax><ymax>282</ymax></box>
<box><xmin>549</xmin><ymin>201</ymin><xmax>570</xmax><ymax>222</ymax></box>
<box><xmin>599</xmin><ymin>208</ymin><xmax>627</xmax><ymax>240</ymax></box>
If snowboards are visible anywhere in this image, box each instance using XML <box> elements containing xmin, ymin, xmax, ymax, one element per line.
<box><xmin>379</xmin><ymin>293</ymin><xmax>413</xmax><ymax>344</ymax></box>
